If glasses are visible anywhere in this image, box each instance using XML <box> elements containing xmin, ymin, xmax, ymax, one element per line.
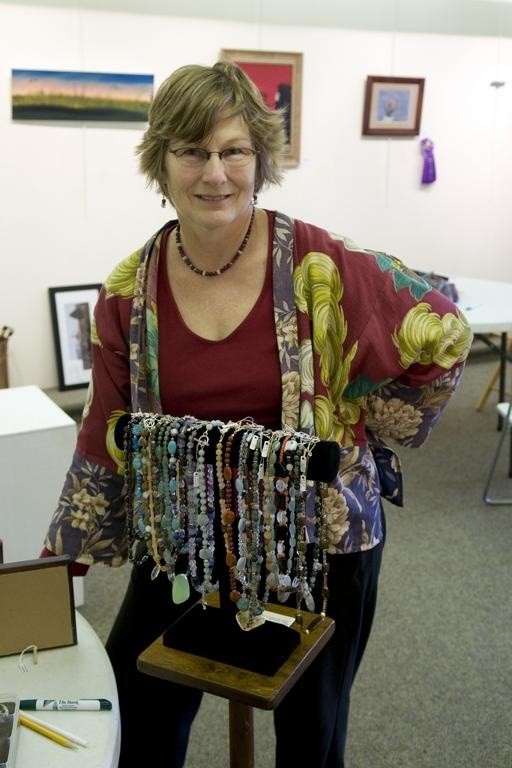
<box><xmin>168</xmin><ymin>145</ymin><xmax>262</xmax><ymax>167</ymax></box>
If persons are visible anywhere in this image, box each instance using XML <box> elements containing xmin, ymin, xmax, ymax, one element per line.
<box><xmin>42</xmin><ymin>58</ymin><xmax>476</xmax><ymax>768</ymax></box>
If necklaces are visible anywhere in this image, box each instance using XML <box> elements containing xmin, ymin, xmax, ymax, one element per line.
<box><xmin>125</xmin><ymin>415</ymin><xmax>329</xmax><ymax>637</ymax></box>
<box><xmin>175</xmin><ymin>207</ymin><xmax>255</xmax><ymax>276</ymax></box>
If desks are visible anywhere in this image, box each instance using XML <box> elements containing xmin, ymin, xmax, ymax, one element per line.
<box><xmin>449</xmin><ymin>276</ymin><xmax>511</xmax><ymax>432</ymax></box>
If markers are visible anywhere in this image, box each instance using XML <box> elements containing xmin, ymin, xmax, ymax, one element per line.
<box><xmin>18</xmin><ymin>697</ymin><xmax>114</xmax><ymax>712</ymax></box>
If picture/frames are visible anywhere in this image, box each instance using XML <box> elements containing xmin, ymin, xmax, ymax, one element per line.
<box><xmin>0</xmin><ymin>553</ymin><xmax>80</xmax><ymax>658</ymax></box>
<box><xmin>217</xmin><ymin>45</ymin><xmax>427</xmax><ymax>170</ymax></box>
<box><xmin>46</xmin><ymin>280</ymin><xmax>107</xmax><ymax>394</ymax></box>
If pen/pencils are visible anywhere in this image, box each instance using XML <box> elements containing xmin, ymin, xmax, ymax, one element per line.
<box><xmin>16</xmin><ymin>709</ymin><xmax>92</xmax><ymax>752</ymax></box>
